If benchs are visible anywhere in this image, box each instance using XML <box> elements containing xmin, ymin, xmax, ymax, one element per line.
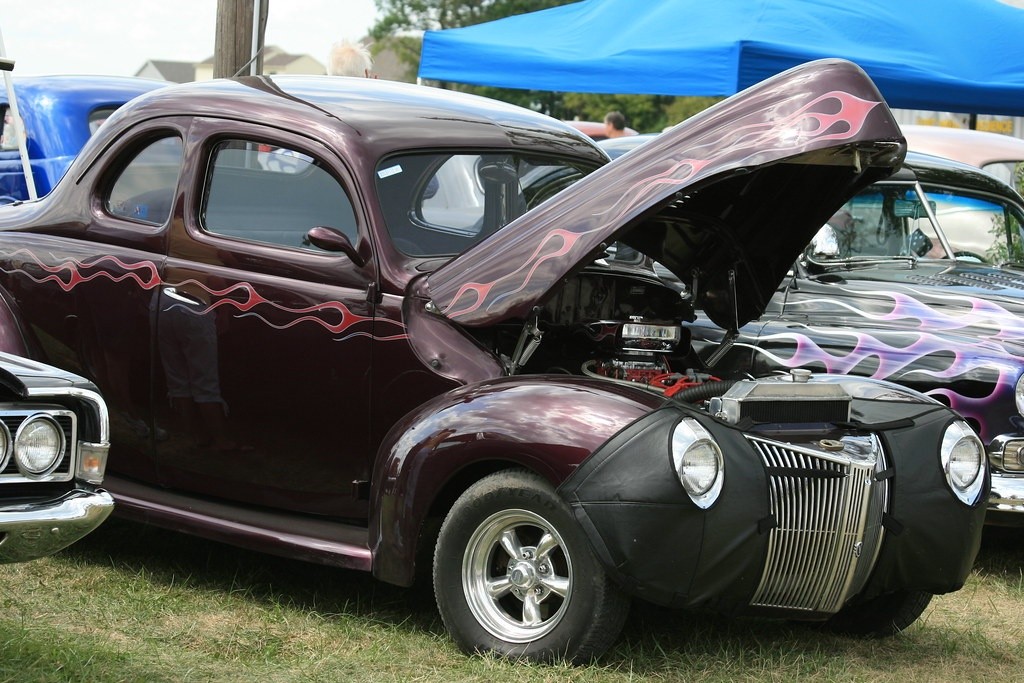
<box><xmin>111</xmin><ymin>189</ymin><xmax>357</xmax><ymax>231</ymax></box>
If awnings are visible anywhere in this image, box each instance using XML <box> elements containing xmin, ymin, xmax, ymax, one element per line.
<box><xmin>419</xmin><ymin>2</ymin><xmax>1024</xmax><ymax>116</ymax></box>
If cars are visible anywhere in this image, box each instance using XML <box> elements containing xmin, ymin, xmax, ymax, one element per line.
<box><xmin>0</xmin><ymin>58</ymin><xmax>990</xmax><ymax>667</ymax></box>
<box><xmin>464</xmin><ymin>133</ymin><xmax>1024</xmax><ymax>540</ymax></box>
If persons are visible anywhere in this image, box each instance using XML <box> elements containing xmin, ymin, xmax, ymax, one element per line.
<box><xmin>603</xmin><ymin>111</ymin><xmax>625</xmax><ymax>139</ymax></box>
